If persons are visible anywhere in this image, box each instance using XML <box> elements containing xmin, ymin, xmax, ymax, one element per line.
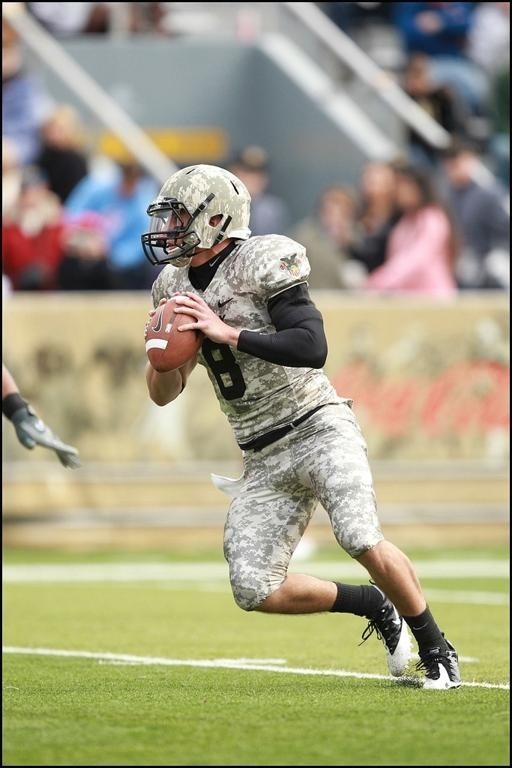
<box><xmin>138</xmin><ymin>157</ymin><xmax>462</xmax><ymax>694</ymax></box>
<box><xmin>1</xmin><ymin>361</ymin><xmax>83</xmax><ymax>472</ymax></box>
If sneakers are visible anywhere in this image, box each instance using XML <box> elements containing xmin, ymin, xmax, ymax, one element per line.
<box><xmin>413</xmin><ymin>632</ymin><xmax>462</xmax><ymax>689</ymax></box>
<box><xmin>359</xmin><ymin>580</ymin><xmax>409</xmax><ymax>677</ymax></box>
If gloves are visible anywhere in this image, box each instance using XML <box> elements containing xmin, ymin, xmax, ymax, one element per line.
<box><xmin>2</xmin><ymin>393</ymin><xmax>82</xmax><ymax>470</ymax></box>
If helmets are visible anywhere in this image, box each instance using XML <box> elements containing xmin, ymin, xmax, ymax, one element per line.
<box><xmin>141</xmin><ymin>164</ymin><xmax>251</xmax><ymax>267</ymax></box>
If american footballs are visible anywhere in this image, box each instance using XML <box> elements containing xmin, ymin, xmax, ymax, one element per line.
<box><xmin>145</xmin><ymin>296</ymin><xmax>204</xmax><ymax>373</ymax></box>
